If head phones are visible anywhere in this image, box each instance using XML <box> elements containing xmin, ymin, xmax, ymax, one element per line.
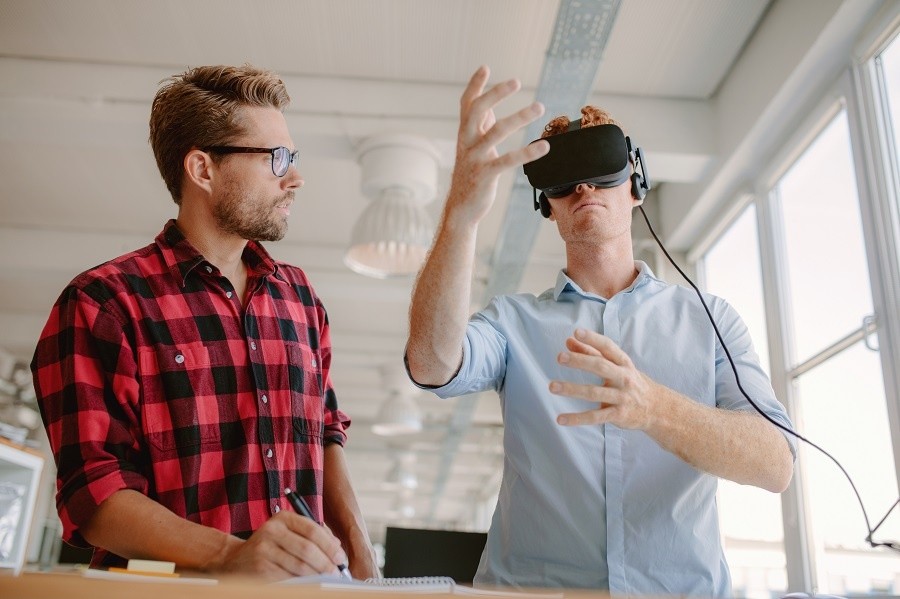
<box><xmin>533</xmin><ymin>147</ymin><xmax>652</xmax><ymax>218</ymax></box>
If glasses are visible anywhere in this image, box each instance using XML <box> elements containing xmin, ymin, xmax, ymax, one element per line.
<box><xmin>199</xmin><ymin>145</ymin><xmax>299</xmax><ymax>177</ymax></box>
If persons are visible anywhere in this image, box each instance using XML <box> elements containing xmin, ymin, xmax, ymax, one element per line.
<box><xmin>403</xmin><ymin>66</ymin><xmax>798</xmax><ymax>598</ymax></box>
<box><xmin>29</xmin><ymin>65</ymin><xmax>382</xmax><ymax>583</ymax></box>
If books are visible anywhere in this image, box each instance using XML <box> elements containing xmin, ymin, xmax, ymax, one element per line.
<box><xmin>277</xmin><ymin>574</ymin><xmax>464</xmax><ymax>594</ymax></box>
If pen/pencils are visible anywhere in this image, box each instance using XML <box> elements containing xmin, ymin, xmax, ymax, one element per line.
<box><xmin>283</xmin><ymin>488</ymin><xmax>353</xmax><ymax>581</ymax></box>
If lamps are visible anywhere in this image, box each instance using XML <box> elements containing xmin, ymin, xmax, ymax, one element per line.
<box><xmin>343</xmin><ymin>140</ymin><xmax>446</xmax><ymax>275</ymax></box>
